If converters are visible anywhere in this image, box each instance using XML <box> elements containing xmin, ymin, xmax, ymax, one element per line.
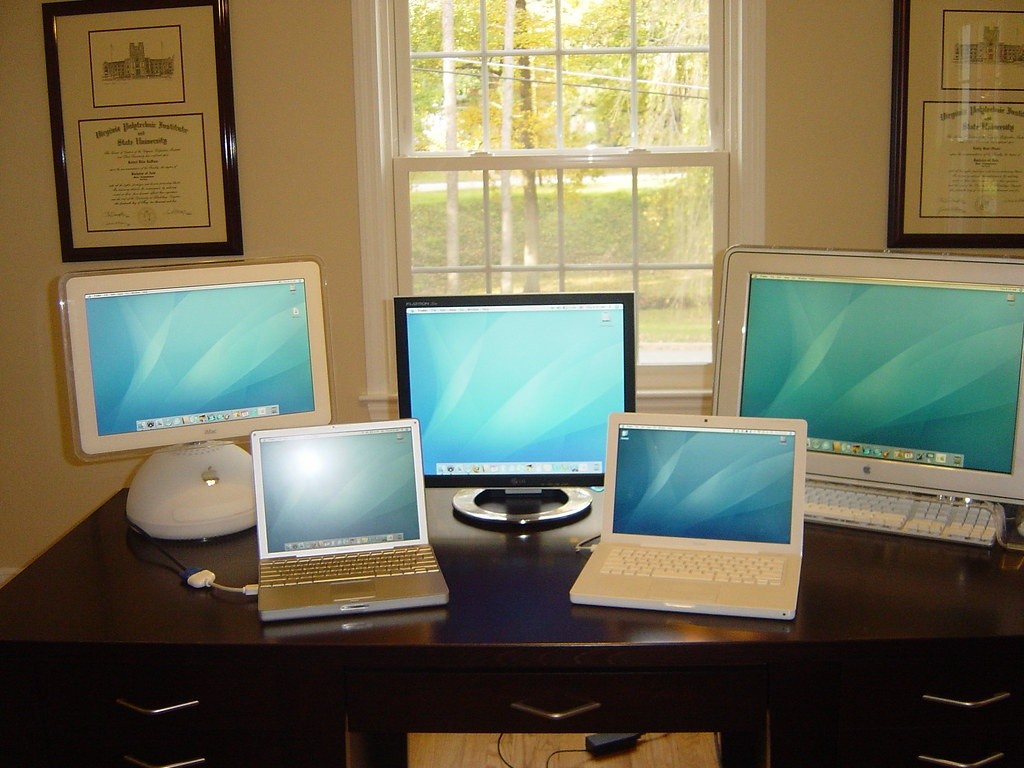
<box><xmin>584</xmin><ymin>731</ymin><xmax>639</xmax><ymax>754</ymax></box>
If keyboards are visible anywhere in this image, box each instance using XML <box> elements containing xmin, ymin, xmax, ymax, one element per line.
<box><xmin>803</xmin><ymin>484</ymin><xmax>998</xmax><ymax>545</ymax></box>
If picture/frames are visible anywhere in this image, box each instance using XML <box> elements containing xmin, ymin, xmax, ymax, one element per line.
<box><xmin>886</xmin><ymin>0</ymin><xmax>1024</xmax><ymax>249</ymax></box>
<box><xmin>42</xmin><ymin>0</ymin><xmax>247</xmax><ymax>265</ymax></box>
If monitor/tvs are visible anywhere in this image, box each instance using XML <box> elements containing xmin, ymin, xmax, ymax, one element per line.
<box><xmin>394</xmin><ymin>290</ymin><xmax>635</xmax><ymax>529</ymax></box>
<box><xmin>58</xmin><ymin>256</ymin><xmax>338</xmax><ymax>537</ymax></box>
<box><xmin>714</xmin><ymin>244</ymin><xmax>1024</xmax><ymax>507</ymax></box>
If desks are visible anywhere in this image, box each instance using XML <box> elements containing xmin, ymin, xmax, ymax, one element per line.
<box><xmin>0</xmin><ymin>488</ymin><xmax>1024</xmax><ymax>768</ymax></box>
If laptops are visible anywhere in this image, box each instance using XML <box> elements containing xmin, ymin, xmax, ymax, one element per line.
<box><xmin>569</xmin><ymin>414</ymin><xmax>809</xmax><ymax>619</ymax></box>
<box><xmin>249</xmin><ymin>418</ymin><xmax>449</xmax><ymax>622</ymax></box>
